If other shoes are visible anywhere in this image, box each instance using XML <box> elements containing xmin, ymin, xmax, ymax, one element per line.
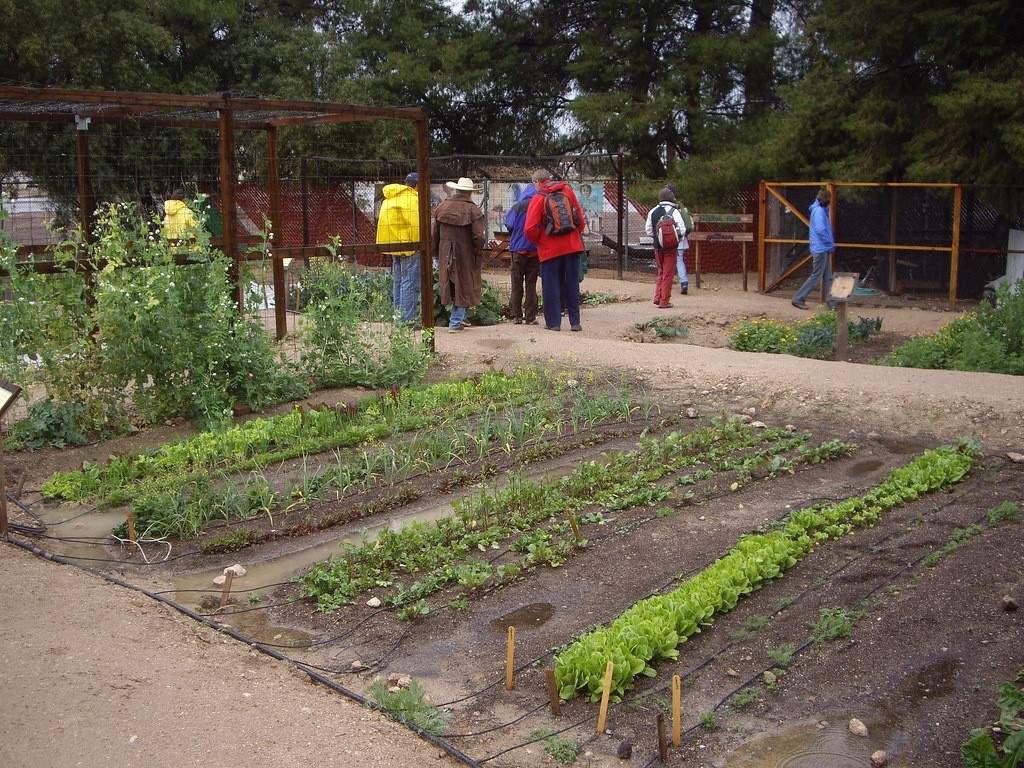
<box><xmin>408</xmin><ymin>322</ymin><xmax>422</xmax><ymax>330</ymax></box>
<box><xmin>526</xmin><ymin>319</ymin><xmax>539</xmax><ymax>325</ymax></box>
<box><xmin>653</xmin><ymin>301</ymin><xmax>673</xmax><ymax>308</ymax></box>
<box><xmin>514</xmin><ymin>316</ymin><xmax>522</xmax><ymax>324</ymax></box>
<box><xmin>792</xmin><ymin>301</ymin><xmax>808</xmax><ymax>310</ymax></box>
<box><xmin>681</xmin><ymin>285</ymin><xmax>687</xmax><ymax>294</ymax></box>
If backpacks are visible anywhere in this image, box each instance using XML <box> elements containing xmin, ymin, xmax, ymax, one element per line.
<box><xmin>537</xmin><ymin>191</ymin><xmax>580</xmax><ymax>236</ymax></box>
<box><xmin>656</xmin><ymin>208</ymin><xmax>680</xmax><ymax>249</ymax></box>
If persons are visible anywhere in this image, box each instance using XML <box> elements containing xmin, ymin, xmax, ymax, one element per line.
<box><xmin>375</xmin><ymin>172</ymin><xmax>422</xmax><ymax>330</ymax></box>
<box><xmin>432</xmin><ymin>177</ymin><xmax>487</xmax><ymax>333</ymax></box>
<box><xmin>162</xmin><ymin>189</ymin><xmax>204</xmax><ymax>254</ymax></box>
<box><xmin>503</xmin><ymin>169</ymin><xmax>586</xmax><ymax>332</ymax></box>
<box><xmin>645</xmin><ymin>182</ymin><xmax>695</xmax><ymax>308</ymax></box>
<box><xmin>791</xmin><ymin>188</ymin><xmax>837</xmax><ymax>310</ymax></box>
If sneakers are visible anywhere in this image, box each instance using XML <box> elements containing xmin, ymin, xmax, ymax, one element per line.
<box><xmin>571</xmin><ymin>325</ymin><xmax>582</xmax><ymax>331</ymax></box>
<box><xmin>544</xmin><ymin>324</ymin><xmax>560</xmax><ymax>330</ymax></box>
<box><xmin>448</xmin><ymin>325</ymin><xmax>464</xmax><ymax>332</ymax></box>
<box><xmin>460</xmin><ymin>318</ymin><xmax>471</xmax><ymax>326</ymax></box>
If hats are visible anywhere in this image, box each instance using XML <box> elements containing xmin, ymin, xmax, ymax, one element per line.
<box><xmin>446</xmin><ymin>177</ymin><xmax>479</xmax><ymax>190</ymax></box>
<box><xmin>406</xmin><ymin>172</ymin><xmax>418</xmax><ymax>182</ymax></box>
<box><xmin>659</xmin><ymin>188</ymin><xmax>674</xmax><ymax>201</ymax></box>
<box><xmin>171</xmin><ymin>189</ymin><xmax>183</xmax><ymax>198</ymax></box>
